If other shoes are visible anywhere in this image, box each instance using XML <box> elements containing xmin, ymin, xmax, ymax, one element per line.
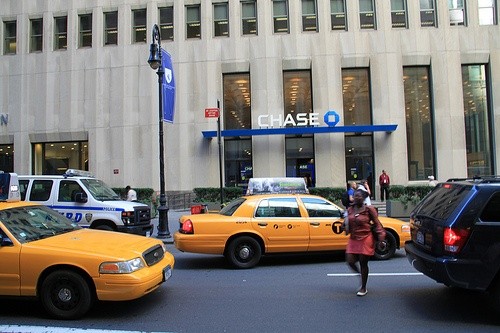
<box><xmin>379</xmin><ymin>200</ymin><xmax>384</xmax><ymax>202</ymax></box>
<box><xmin>356</xmin><ymin>289</ymin><xmax>368</xmax><ymax>296</ymax></box>
<box><xmin>355</xmin><ymin>284</ymin><xmax>363</xmax><ymax>292</ymax></box>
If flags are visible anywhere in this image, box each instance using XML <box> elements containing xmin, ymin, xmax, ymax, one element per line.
<box><xmin>161</xmin><ymin>48</ymin><xmax>176</xmax><ymax>124</ymax></box>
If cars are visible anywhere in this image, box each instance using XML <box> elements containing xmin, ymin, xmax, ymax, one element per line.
<box><xmin>174</xmin><ymin>177</ymin><xmax>413</xmax><ymax>269</ymax></box>
<box><xmin>0</xmin><ymin>172</ymin><xmax>174</xmax><ymax>322</ymax></box>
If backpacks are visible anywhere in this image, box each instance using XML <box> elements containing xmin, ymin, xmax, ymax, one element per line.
<box><xmin>339</xmin><ymin>189</ymin><xmax>355</xmax><ymax>209</ymax></box>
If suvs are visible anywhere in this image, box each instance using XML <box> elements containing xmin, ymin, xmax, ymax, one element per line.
<box><xmin>0</xmin><ymin>169</ymin><xmax>154</xmax><ymax>236</ymax></box>
<box><xmin>405</xmin><ymin>176</ymin><xmax>500</xmax><ymax>310</ymax></box>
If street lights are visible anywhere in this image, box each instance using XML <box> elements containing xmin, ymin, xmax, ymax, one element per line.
<box><xmin>148</xmin><ymin>24</ymin><xmax>173</xmax><ymax>238</ymax></box>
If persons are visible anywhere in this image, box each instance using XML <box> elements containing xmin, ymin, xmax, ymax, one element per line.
<box><xmin>379</xmin><ymin>169</ymin><xmax>390</xmax><ymax>202</ymax></box>
<box><xmin>341</xmin><ymin>189</ymin><xmax>386</xmax><ymax>296</ymax></box>
<box><xmin>356</xmin><ymin>178</ymin><xmax>371</xmax><ymax>205</ymax></box>
<box><xmin>346</xmin><ymin>180</ymin><xmax>357</xmax><ymax>209</ymax></box>
<box><xmin>126</xmin><ymin>186</ymin><xmax>137</xmax><ymax>202</ymax></box>
